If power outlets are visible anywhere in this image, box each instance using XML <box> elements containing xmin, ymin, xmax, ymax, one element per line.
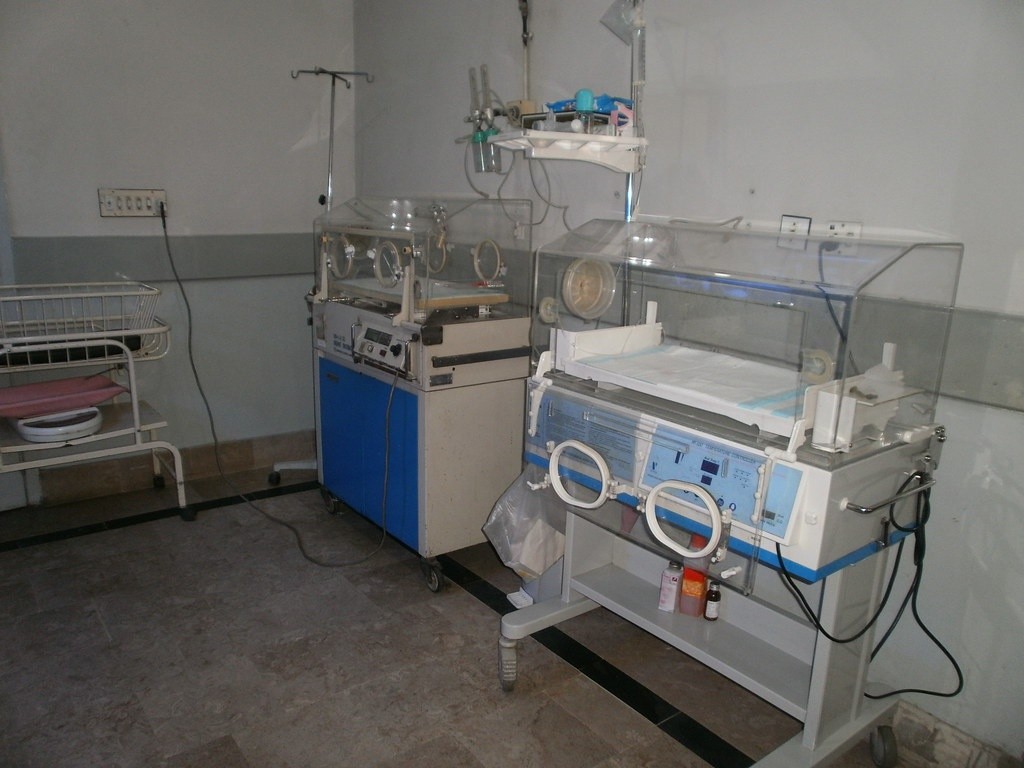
<box><xmin>825</xmin><ymin>222</ymin><xmax>864</xmax><ymax>256</ymax></box>
<box><xmin>99</xmin><ymin>189</ymin><xmax>168</xmax><ymax>218</ymax></box>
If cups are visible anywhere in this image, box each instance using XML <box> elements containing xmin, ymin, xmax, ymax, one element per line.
<box><xmin>574</xmin><ymin>89</ymin><xmax>594</xmax><ymax>112</ymax></box>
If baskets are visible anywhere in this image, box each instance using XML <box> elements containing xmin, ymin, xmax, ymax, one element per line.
<box><xmin>0</xmin><ymin>281</ymin><xmax>161</xmax><ymax>370</ymax></box>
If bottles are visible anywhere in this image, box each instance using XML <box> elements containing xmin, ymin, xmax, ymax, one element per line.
<box><xmin>704</xmin><ymin>581</ymin><xmax>721</xmax><ymax>621</ymax></box>
<box><xmin>658</xmin><ymin>560</ymin><xmax>683</xmax><ymax>612</ymax></box>
<box><xmin>678</xmin><ymin>533</ymin><xmax>711</xmax><ymax>616</ymax></box>
